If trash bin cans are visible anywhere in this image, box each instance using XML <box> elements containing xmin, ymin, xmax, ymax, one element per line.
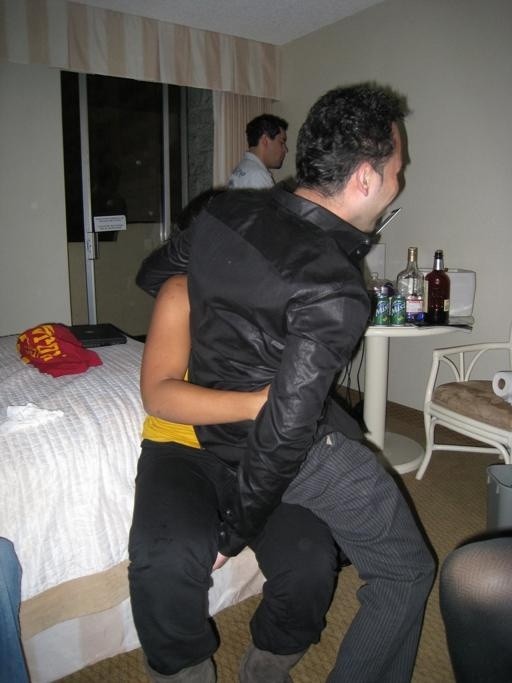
<box><xmin>486</xmin><ymin>464</ymin><xmax>511</xmax><ymax>537</ymax></box>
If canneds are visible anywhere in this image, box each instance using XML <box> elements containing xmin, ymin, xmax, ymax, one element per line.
<box><xmin>390</xmin><ymin>296</ymin><xmax>406</xmax><ymax>328</ymax></box>
<box><xmin>373</xmin><ymin>294</ymin><xmax>389</xmax><ymax>327</ymax></box>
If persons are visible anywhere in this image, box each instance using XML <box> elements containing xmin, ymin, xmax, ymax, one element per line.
<box><xmin>221</xmin><ymin>113</ymin><xmax>289</xmax><ymax>190</ymax></box>
<box><xmin>436</xmin><ymin>533</ymin><xmax>512</xmax><ymax>683</ymax></box>
<box><xmin>132</xmin><ymin>80</ymin><xmax>442</xmax><ymax>682</ymax></box>
<box><xmin>126</xmin><ymin>175</ymin><xmax>342</xmax><ymax>683</ymax></box>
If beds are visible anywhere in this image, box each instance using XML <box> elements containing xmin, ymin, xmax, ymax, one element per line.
<box><xmin>0</xmin><ymin>320</ymin><xmax>277</xmax><ymax>669</ymax></box>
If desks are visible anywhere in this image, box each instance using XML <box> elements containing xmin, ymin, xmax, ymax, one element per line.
<box><xmin>346</xmin><ymin>316</ymin><xmax>466</xmax><ymax>478</ymax></box>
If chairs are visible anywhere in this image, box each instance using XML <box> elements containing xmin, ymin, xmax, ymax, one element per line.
<box><xmin>412</xmin><ymin>341</ymin><xmax>510</xmax><ymax>481</ymax></box>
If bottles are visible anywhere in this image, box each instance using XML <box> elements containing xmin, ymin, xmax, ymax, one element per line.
<box><xmin>396</xmin><ymin>246</ymin><xmax>450</xmax><ymax>324</ymax></box>
